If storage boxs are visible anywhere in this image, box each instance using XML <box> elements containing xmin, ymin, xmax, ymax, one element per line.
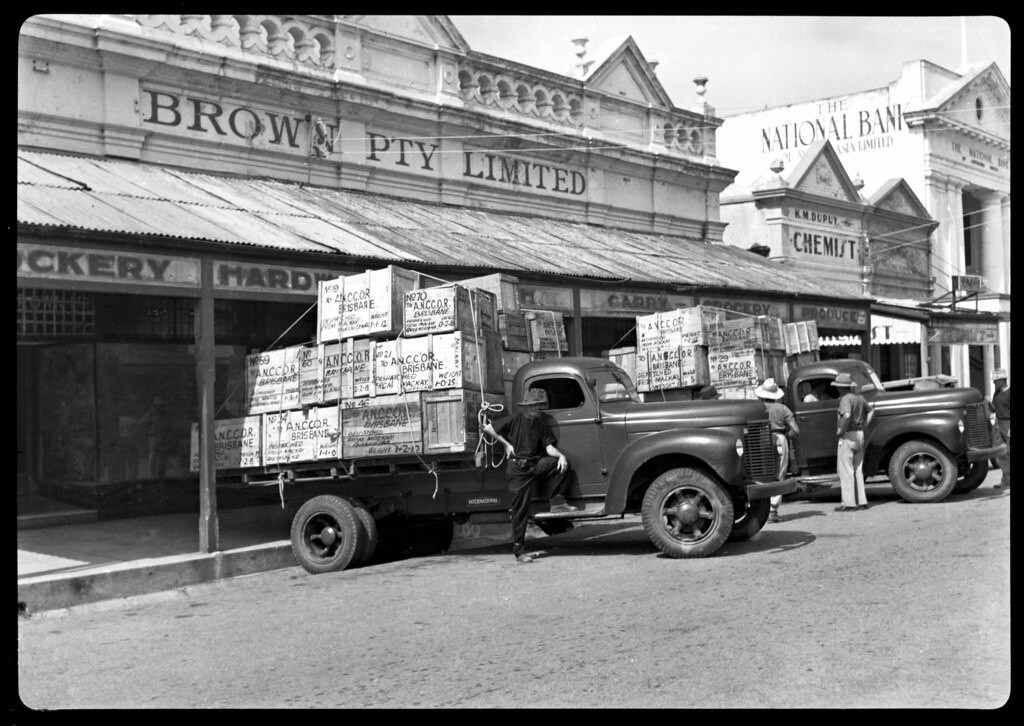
<box><xmin>191</xmin><ymin>266</ymin><xmax>568</xmax><ymax>472</ymax></box>
<box><xmin>602</xmin><ymin>307</ymin><xmax>819</xmax><ymax>402</ymax></box>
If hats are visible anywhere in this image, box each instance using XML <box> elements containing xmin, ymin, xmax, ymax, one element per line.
<box><xmin>830</xmin><ymin>373</ymin><xmax>858</xmax><ymax>387</ymax></box>
<box><xmin>991</xmin><ymin>368</ymin><xmax>1009</xmax><ymax>382</ymax></box>
<box><xmin>755</xmin><ymin>377</ymin><xmax>784</xmax><ymax>399</ymax></box>
<box><xmin>516</xmin><ymin>389</ymin><xmax>547</xmax><ymax>406</ymax></box>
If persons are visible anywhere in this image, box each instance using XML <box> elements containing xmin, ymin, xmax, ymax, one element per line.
<box><xmin>831</xmin><ymin>372</ymin><xmax>875</xmax><ymax>511</ymax></box>
<box><xmin>483</xmin><ymin>387</ymin><xmax>576</xmax><ymax>563</ymax></box>
<box><xmin>755</xmin><ymin>378</ymin><xmax>799</xmax><ymax>522</ymax></box>
<box><xmin>804</xmin><ymin>379</ymin><xmax>834</xmax><ymax>404</ymax></box>
<box><xmin>985</xmin><ymin>367</ymin><xmax>1010</xmax><ymax>488</ymax></box>
<box><xmin>698</xmin><ymin>386</ymin><xmax>723</xmax><ymax>400</ymax></box>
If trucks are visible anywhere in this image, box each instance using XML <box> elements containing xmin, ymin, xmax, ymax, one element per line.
<box><xmin>215</xmin><ymin>354</ymin><xmax>800</xmax><ymax>574</ymax></box>
<box><xmin>778</xmin><ymin>358</ymin><xmax>1006</xmax><ymax>505</ymax></box>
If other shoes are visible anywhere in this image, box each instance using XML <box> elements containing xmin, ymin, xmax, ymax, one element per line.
<box><xmin>517</xmin><ymin>554</ymin><xmax>533</xmax><ymax>564</ymax></box>
<box><xmin>859</xmin><ymin>504</ymin><xmax>867</xmax><ymax>511</ymax></box>
<box><xmin>767</xmin><ymin>513</ymin><xmax>786</xmax><ymax>523</ymax></box>
<box><xmin>835</xmin><ymin>504</ymin><xmax>857</xmax><ymax>512</ymax></box>
<box><xmin>550</xmin><ymin>502</ymin><xmax>578</xmax><ymax>513</ymax></box>
<box><xmin>994</xmin><ymin>480</ymin><xmax>1011</xmax><ymax>488</ymax></box>
